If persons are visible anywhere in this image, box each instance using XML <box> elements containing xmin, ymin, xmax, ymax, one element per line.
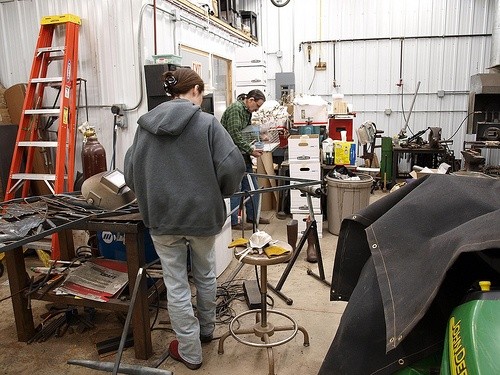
<box><xmin>220</xmin><ymin>90</ymin><xmax>270</xmax><ymax>230</ymax></box>
<box><xmin>123</xmin><ymin>67</ymin><xmax>247</xmax><ymax>370</ymax></box>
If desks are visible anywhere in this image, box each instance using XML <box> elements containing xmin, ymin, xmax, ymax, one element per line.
<box><xmin>322</xmin><ymin>164</ymin><xmax>357</xmax><ymax>172</ymax></box>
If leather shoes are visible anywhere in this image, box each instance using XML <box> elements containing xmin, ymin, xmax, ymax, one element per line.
<box><xmin>169</xmin><ymin>340</ymin><xmax>202</xmax><ymax>369</ymax></box>
<box><xmin>200</xmin><ymin>334</ymin><xmax>212</xmax><ymax>341</ymax></box>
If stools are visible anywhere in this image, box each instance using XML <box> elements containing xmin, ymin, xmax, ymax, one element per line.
<box><xmin>218</xmin><ymin>241</ymin><xmax>309</xmax><ymax>375</ymax></box>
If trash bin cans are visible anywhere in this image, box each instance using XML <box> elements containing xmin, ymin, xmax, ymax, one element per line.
<box><xmin>325</xmin><ymin>171</ymin><xmax>374</xmax><ymax>237</ymax></box>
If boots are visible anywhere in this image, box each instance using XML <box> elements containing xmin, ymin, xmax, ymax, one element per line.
<box><xmin>307</xmin><ymin>221</ymin><xmax>317</xmax><ymax>263</ymax></box>
<box><xmin>287</xmin><ymin>220</ymin><xmax>298</xmax><ymax>252</ymax></box>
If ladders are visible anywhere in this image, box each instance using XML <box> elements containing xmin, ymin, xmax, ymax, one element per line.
<box><xmin>0</xmin><ymin>14</ymin><xmax>83</xmax><ymax>281</ymax></box>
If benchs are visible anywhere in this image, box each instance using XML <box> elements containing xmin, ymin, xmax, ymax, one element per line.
<box><xmin>357</xmin><ymin>167</ymin><xmax>380</xmax><ymax>195</ymax></box>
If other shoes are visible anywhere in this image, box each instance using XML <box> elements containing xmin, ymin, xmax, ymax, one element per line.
<box><xmin>246</xmin><ymin>216</ymin><xmax>271</xmax><ymax>223</ymax></box>
<box><xmin>231</xmin><ymin>223</ymin><xmax>252</xmax><ymax>230</ymax></box>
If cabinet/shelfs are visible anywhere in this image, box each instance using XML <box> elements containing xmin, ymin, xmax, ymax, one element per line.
<box><xmin>6</xmin><ymin>195</ymin><xmax>165</xmax><ymax>360</ymax></box>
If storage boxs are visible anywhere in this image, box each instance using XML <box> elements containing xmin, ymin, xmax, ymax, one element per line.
<box><xmin>288</xmin><ymin>135</ymin><xmax>323</xmax><ymax>239</ymax></box>
<box><xmin>334</xmin><ymin>140</ymin><xmax>356</xmax><ymax>165</ymax></box>
<box><xmin>241</xmin><ymin>123</ymin><xmax>280</xmax><ymax>152</ymax></box>
<box><xmin>293</xmin><ymin>106</ymin><xmax>328</xmax><ymax>126</ymax></box>
<box><xmin>322</xmin><ymin>142</ymin><xmax>334</xmax><ymax>161</ymax></box>
<box><xmin>243</xmin><ymin>280</ymin><xmax>262</xmax><ymax>310</ymax></box>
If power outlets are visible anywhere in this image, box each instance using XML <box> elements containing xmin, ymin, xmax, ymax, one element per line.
<box><xmin>113</xmin><ymin>104</ymin><xmax>128</xmax><ymax>128</ymax></box>
